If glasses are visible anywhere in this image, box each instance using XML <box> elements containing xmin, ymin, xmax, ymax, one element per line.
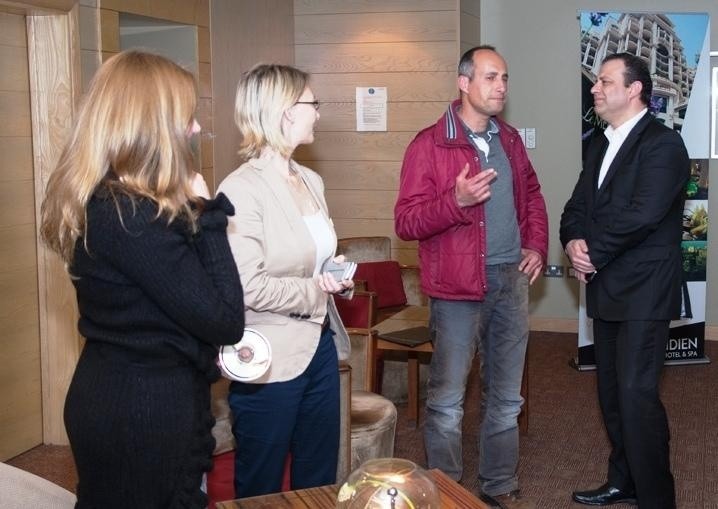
<box><xmin>295</xmin><ymin>99</ymin><xmax>322</xmax><ymax>110</ymax></box>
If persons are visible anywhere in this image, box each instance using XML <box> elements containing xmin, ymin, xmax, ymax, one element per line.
<box><xmin>559</xmin><ymin>53</ymin><xmax>690</xmax><ymax>509</ymax></box>
<box><xmin>39</xmin><ymin>50</ymin><xmax>245</xmax><ymax>508</ymax></box>
<box><xmin>393</xmin><ymin>46</ymin><xmax>549</xmax><ymax>509</ymax></box>
<box><xmin>215</xmin><ymin>64</ymin><xmax>355</xmax><ymax>500</ymax></box>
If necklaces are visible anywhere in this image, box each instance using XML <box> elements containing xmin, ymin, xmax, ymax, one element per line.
<box><xmin>283</xmin><ymin>174</ymin><xmax>316</xmax><ymax>213</ymax></box>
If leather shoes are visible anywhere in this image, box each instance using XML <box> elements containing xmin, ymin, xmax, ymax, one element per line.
<box><xmin>573</xmin><ymin>482</ymin><xmax>638</xmax><ymax>506</ymax></box>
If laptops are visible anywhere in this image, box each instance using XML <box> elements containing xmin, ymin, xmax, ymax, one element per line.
<box><xmin>382</xmin><ymin>327</ymin><xmax>432</xmax><ymax>348</ymax></box>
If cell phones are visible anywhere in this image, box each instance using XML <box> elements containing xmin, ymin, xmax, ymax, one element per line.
<box><xmin>322</xmin><ymin>261</ymin><xmax>358</xmax><ymax>282</ymax></box>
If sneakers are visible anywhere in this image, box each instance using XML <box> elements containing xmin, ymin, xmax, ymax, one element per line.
<box><xmin>480</xmin><ymin>490</ymin><xmax>536</xmax><ymax>509</ymax></box>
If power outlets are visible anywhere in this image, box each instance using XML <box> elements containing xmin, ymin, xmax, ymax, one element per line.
<box><xmin>568</xmin><ymin>267</ymin><xmax>576</xmax><ymax>278</ymax></box>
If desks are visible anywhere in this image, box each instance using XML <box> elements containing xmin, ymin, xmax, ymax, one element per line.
<box><xmin>214</xmin><ymin>468</ymin><xmax>493</xmax><ymax>509</ymax></box>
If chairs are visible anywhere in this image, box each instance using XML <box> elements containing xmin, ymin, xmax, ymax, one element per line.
<box><xmin>334</xmin><ymin>236</ymin><xmax>432</xmax><ymax>405</ymax></box>
<box><xmin>200</xmin><ymin>361</ymin><xmax>352</xmax><ymax>502</ymax></box>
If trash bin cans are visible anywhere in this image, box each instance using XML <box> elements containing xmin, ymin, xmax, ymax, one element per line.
<box><xmin>349</xmin><ymin>390</ymin><xmax>397</xmax><ymax>475</ymax></box>
